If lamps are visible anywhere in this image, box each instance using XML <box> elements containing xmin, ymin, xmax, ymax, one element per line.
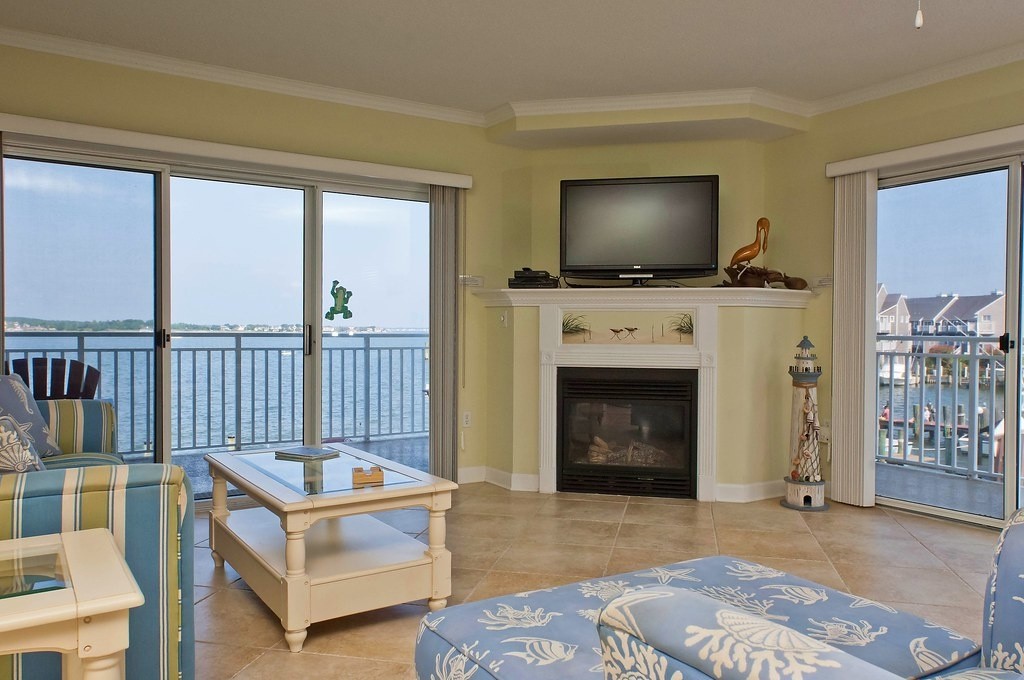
<box><xmin>915</xmin><ymin>0</ymin><xmax>923</xmax><ymax>30</ymax></box>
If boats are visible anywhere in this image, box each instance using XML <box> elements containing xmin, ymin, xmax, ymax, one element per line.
<box><xmin>884</xmin><ymin>437</ymin><xmax>913</xmax><ymax>456</ymax></box>
<box><xmin>880</xmin><ymin>361</ymin><xmax>917</xmax><ymax>386</ymax></box>
<box><xmin>957</xmin><ymin>418</ymin><xmax>1002</xmax><ymax>455</ymax></box>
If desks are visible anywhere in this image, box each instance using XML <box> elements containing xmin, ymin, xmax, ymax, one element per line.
<box><xmin>0</xmin><ymin>527</ymin><xmax>146</xmax><ymax>680</ymax></box>
<box><xmin>202</xmin><ymin>441</ymin><xmax>459</xmax><ymax>653</ymax></box>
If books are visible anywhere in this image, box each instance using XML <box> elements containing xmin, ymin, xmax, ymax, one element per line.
<box><xmin>275</xmin><ymin>447</ymin><xmax>340</xmax><ymax>460</ymax></box>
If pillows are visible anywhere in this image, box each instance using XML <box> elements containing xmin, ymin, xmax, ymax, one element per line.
<box><xmin>0</xmin><ymin>373</ymin><xmax>64</xmax><ymax>459</ymax></box>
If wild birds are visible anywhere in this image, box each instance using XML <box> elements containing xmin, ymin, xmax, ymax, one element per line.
<box><xmin>730</xmin><ymin>218</ymin><xmax>770</xmax><ymax>267</ymax></box>
<box><xmin>610</xmin><ymin>327</ymin><xmax>638</xmax><ymax>340</ymax></box>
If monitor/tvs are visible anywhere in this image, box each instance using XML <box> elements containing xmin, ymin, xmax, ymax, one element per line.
<box><xmin>560</xmin><ymin>174</ymin><xmax>719</xmax><ymax>288</ymax></box>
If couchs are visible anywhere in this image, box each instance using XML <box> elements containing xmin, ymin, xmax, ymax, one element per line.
<box><xmin>0</xmin><ymin>373</ymin><xmax>195</xmax><ymax>680</ymax></box>
<box><xmin>411</xmin><ymin>507</ymin><xmax>1024</xmax><ymax>680</ymax></box>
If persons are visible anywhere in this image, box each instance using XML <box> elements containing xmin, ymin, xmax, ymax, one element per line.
<box><xmin>924</xmin><ymin>407</ymin><xmax>930</xmax><ymax>422</ymax></box>
<box><xmin>882</xmin><ymin>406</ymin><xmax>889</xmax><ymax>419</ymax></box>
